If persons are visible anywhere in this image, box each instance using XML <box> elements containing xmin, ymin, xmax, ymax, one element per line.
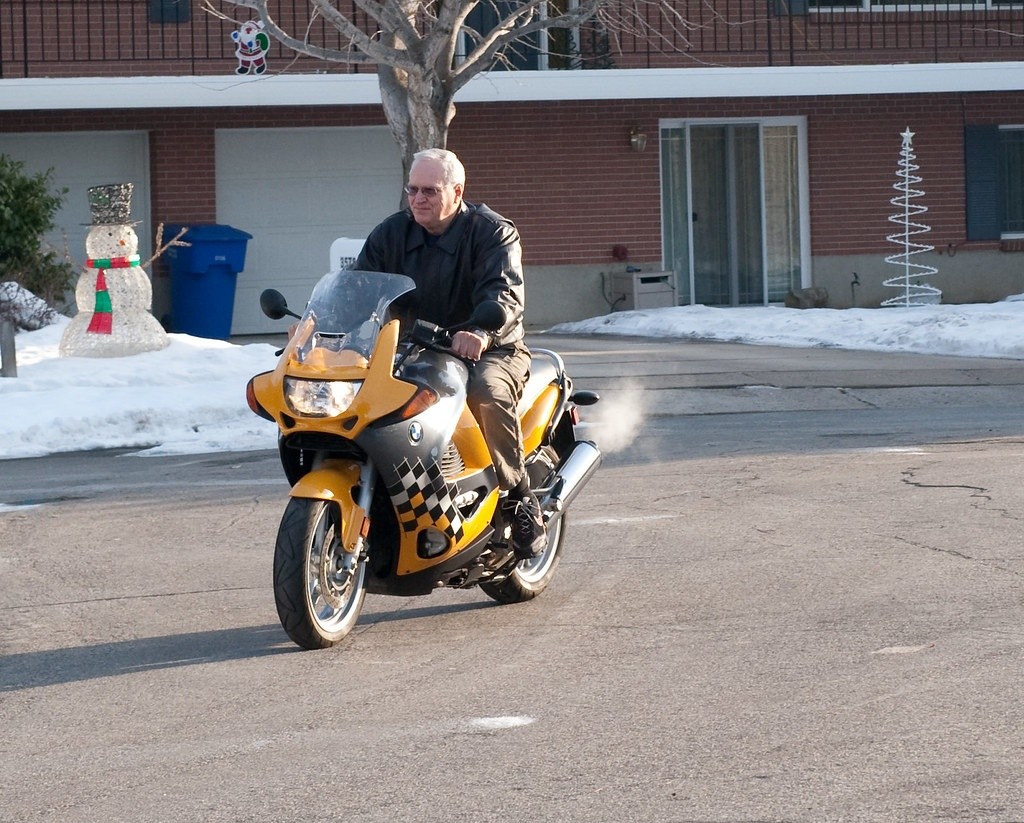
<box><xmin>319</xmin><ymin>147</ymin><xmax>550</xmax><ymax>559</ymax></box>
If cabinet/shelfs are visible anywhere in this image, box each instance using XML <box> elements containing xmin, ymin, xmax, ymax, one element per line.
<box><xmin>610</xmin><ymin>269</ymin><xmax>679</xmax><ymax>311</ymax></box>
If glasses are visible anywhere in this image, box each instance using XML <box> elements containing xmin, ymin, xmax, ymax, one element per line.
<box><xmin>403</xmin><ymin>185</ymin><xmax>457</xmax><ymax>197</ymax></box>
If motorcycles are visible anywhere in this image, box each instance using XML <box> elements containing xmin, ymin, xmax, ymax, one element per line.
<box><xmin>245</xmin><ymin>270</ymin><xmax>602</xmax><ymax>651</ymax></box>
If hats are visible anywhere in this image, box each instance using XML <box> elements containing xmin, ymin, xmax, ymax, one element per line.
<box><xmin>79</xmin><ymin>183</ymin><xmax>143</xmax><ymax>227</ymax></box>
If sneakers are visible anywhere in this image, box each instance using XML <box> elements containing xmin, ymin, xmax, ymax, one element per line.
<box><xmin>502</xmin><ymin>493</ymin><xmax>548</xmax><ymax>561</ymax></box>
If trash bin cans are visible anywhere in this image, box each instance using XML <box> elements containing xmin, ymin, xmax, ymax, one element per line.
<box><xmin>164</xmin><ymin>224</ymin><xmax>254</xmax><ymax>340</ymax></box>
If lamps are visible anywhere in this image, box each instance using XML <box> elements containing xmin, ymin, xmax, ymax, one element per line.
<box><xmin>627</xmin><ymin>121</ymin><xmax>649</xmax><ymax>153</ymax></box>
